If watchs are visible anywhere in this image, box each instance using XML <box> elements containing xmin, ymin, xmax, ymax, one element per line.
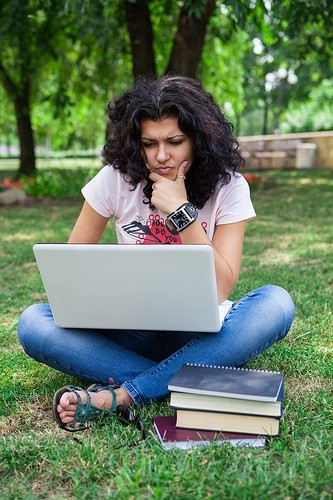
<box><xmin>167</xmin><ymin>201</ymin><xmax>199</xmax><ymax>232</ymax></box>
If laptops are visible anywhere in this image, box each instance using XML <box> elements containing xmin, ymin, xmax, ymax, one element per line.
<box><xmin>32</xmin><ymin>243</ymin><xmax>233</xmax><ymax>332</ymax></box>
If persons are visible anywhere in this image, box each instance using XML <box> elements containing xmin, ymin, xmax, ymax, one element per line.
<box><xmin>18</xmin><ymin>77</ymin><xmax>294</xmax><ymax>432</ymax></box>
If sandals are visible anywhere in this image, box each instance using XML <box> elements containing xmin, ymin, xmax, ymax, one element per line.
<box><xmin>53</xmin><ymin>383</ymin><xmax>120</xmax><ymax>431</ymax></box>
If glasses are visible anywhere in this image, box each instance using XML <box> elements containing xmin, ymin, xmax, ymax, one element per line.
<box><xmin>115</xmin><ymin>406</ymin><xmax>145</xmax><ymax>447</ymax></box>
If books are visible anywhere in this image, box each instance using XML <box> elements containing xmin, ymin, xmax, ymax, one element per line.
<box><xmin>152</xmin><ymin>364</ymin><xmax>285</xmax><ymax>453</ymax></box>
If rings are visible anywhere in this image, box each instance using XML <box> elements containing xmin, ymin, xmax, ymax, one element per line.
<box><xmin>177</xmin><ymin>173</ymin><xmax>187</xmax><ymax>183</ymax></box>
